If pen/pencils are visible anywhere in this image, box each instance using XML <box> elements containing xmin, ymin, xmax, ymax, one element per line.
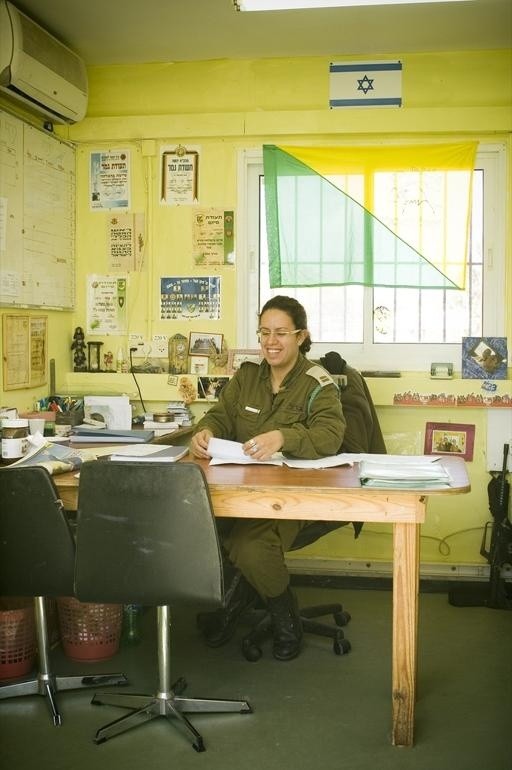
<box><xmin>36</xmin><ymin>397</ymin><xmax>83</xmax><ymax>411</ymax></box>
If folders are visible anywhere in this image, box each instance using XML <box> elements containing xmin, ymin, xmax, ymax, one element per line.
<box><xmin>112</xmin><ymin>445</ymin><xmax>189</xmax><ymax>462</ymax></box>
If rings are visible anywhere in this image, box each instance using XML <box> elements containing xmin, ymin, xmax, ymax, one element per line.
<box><xmin>249</xmin><ymin>439</ymin><xmax>256</xmax><ymax>447</ymax></box>
<box><xmin>251</xmin><ymin>445</ymin><xmax>258</xmax><ymax>454</ymax></box>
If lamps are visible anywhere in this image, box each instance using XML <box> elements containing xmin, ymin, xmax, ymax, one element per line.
<box><xmin>232</xmin><ymin>1</ymin><xmax>432</xmax><ymax>11</ymax></box>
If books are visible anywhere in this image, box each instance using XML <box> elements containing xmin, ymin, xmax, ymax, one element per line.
<box><xmin>110</xmin><ymin>442</ymin><xmax>189</xmax><ymax>463</ymax></box>
<box><xmin>166</xmin><ymin>402</ymin><xmax>192</xmax><ymax>427</ymax></box>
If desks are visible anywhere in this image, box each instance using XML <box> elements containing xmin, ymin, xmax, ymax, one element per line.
<box><xmin>0</xmin><ymin>421</ymin><xmax>472</xmax><ymax>748</ymax></box>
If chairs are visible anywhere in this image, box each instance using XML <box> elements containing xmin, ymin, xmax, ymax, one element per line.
<box><xmin>72</xmin><ymin>459</ymin><xmax>254</xmax><ymax>752</ymax></box>
<box><xmin>0</xmin><ymin>466</ymin><xmax>129</xmax><ymax>727</ymax></box>
<box><xmin>196</xmin><ymin>358</ymin><xmax>369</xmax><ymax>663</ymax></box>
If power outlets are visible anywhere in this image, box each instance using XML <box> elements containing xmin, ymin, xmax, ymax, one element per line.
<box><xmin>127</xmin><ymin>333</ymin><xmax>146</xmax><ymax>358</ymax></box>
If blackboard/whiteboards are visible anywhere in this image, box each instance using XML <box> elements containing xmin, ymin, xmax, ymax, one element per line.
<box><xmin>0</xmin><ymin>105</ymin><xmax>77</xmax><ymax>313</ymax></box>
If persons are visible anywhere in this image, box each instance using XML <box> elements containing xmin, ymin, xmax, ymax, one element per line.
<box><xmin>191</xmin><ymin>294</ymin><xmax>346</xmax><ymax>660</ymax></box>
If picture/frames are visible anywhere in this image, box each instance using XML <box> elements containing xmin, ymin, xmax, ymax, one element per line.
<box><xmin>188</xmin><ymin>332</ymin><xmax>224</xmax><ymax>357</ymax></box>
<box><xmin>423</xmin><ymin>421</ymin><xmax>476</xmax><ymax>463</ymax></box>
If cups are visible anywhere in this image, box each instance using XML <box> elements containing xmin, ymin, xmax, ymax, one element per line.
<box><xmin>28</xmin><ymin>419</ymin><xmax>46</xmax><ymax>437</ymax></box>
<box><xmin>56</xmin><ymin>409</ymin><xmax>84</xmax><ymax>425</ymax></box>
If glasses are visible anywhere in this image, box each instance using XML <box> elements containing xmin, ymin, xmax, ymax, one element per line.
<box><xmin>256</xmin><ymin>329</ymin><xmax>301</xmax><ymax>342</ymax></box>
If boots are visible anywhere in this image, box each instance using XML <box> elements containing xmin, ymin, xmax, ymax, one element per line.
<box><xmin>206</xmin><ymin>577</ymin><xmax>248</xmax><ymax>647</ymax></box>
<box><xmin>266</xmin><ymin>575</ymin><xmax>303</xmax><ymax>661</ymax></box>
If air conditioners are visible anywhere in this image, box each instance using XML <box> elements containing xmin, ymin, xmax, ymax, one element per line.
<box><xmin>0</xmin><ymin>0</ymin><xmax>89</xmax><ymax>127</ymax></box>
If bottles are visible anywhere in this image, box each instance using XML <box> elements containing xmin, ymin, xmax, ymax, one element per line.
<box><xmin>123</xmin><ymin>604</ymin><xmax>141</xmax><ymax>646</ymax></box>
<box><xmin>1</xmin><ymin>419</ymin><xmax>28</xmax><ymax>465</ymax></box>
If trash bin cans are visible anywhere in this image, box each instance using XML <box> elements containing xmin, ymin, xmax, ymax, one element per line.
<box><xmin>61</xmin><ymin>596</ymin><xmax>126</xmax><ymax>663</ymax></box>
<box><xmin>0</xmin><ymin>606</ymin><xmax>39</xmax><ymax>678</ymax></box>
